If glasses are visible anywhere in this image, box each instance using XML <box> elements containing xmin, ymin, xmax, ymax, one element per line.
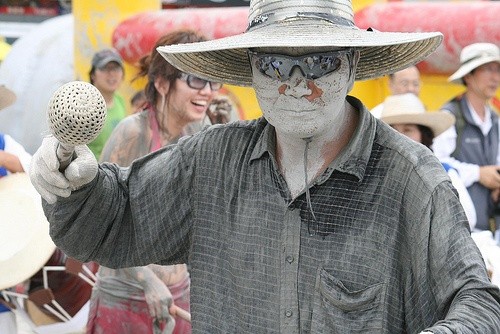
<box><xmin>242</xmin><ymin>49</ymin><xmax>353</xmax><ymax>81</ymax></box>
<box><xmin>177</xmin><ymin>73</ymin><xmax>224</xmax><ymax>90</ymax></box>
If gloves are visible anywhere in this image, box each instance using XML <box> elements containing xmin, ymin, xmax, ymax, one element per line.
<box><xmin>28</xmin><ymin>134</ymin><xmax>99</xmax><ymax>204</ymax></box>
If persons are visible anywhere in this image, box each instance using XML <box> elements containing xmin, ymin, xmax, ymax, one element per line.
<box><xmin>0</xmin><ymin>27</ymin><xmax>500</xmax><ymax>334</ymax></box>
<box><xmin>27</xmin><ymin>0</ymin><xmax>500</xmax><ymax>334</ymax></box>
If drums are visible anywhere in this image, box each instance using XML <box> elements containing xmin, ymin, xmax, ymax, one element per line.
<box><xmin>0</xmin><ymin>169</ymin><xmax>101</xmax><ymax>334</ymax></box>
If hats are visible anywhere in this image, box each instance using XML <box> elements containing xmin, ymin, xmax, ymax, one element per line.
<box><xmin>370</xmin><ymin>93</ymin><xmax>456</xmax><ymax>139</ymax></box>
<box><xmin>156</xmin><ymin>0</ymin><xmax>445</xmax><ymax>80</ymax></box>
<box><xmin>92</xmin><ymin>50</ymin><xmax>125</xmax><ymax>71</ymax></box>
<box><xmin>446</xmin><ymin>43</ymin><xmax>500</xmax><ymax>82</ymax></box>
